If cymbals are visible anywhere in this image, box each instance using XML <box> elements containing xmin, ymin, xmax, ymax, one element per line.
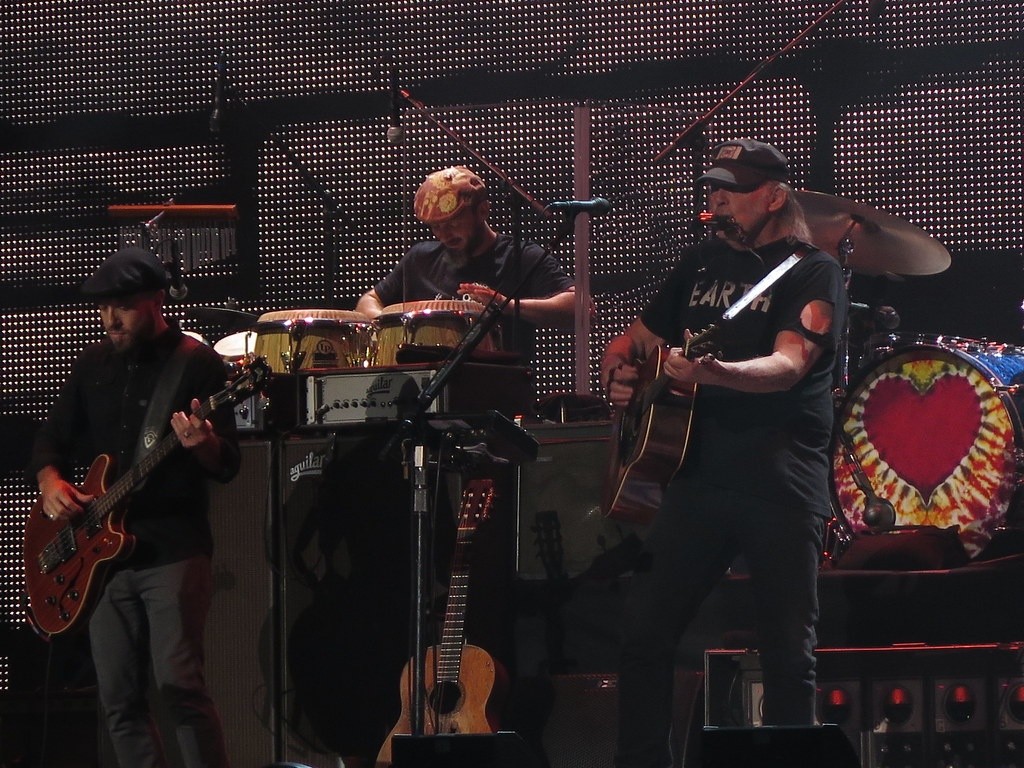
<box><xmin>184</xmin><ymin>305</ymin><xmax>260</xmax><ymax>330</ymax></box>
<box><xmin>794</xmin><ymin>187</ymin><xmax>956</xmax><ymax>277</ymax></box>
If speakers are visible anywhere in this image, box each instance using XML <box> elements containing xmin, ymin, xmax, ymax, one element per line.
<box><xmin>540</xmin><ymin>669</ymin><xmax>705</xmax><ymax>768</ymax></box>
<box><xmin>98</xmin><ymin>433</ymin><xmax>383</xmax><ymax>765</ymax></box>
<box><xmin>514</xmin><ymin>421</ymin><xmax>747</xmax><ymax>687</ymax></box>
<box><xmin>390</xmin><ymin>731</ymin><xmax>542</xmax><ymax>768</ymax></box>
<box><xmin>703</xmin><ymin>725</ymin><xmax>861</xmax><ymax>768</ymax></box>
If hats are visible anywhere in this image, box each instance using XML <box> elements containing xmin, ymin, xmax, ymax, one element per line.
<box><xmin>82</xmin><ymin>248</ymin><xmax>168</xmax><ymax>301</ymax></box>
<box><xmin>696</xmin><ymin>140</ymin><xmax>788</xmax><ymax>193</ymax></box>
<box><xmin>413</xmin><ymin>166</ymin><xmax>489</xmax><ymax>223</ymax></box>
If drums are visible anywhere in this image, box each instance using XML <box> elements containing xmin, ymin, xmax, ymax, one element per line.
<box><xmin>373</xmin><ymin>299</ymin><xmax>496</xmax><ymax>365</ymax></box>
<box><xmin>255</xmin><ymin>308</ymin><xmax>373</xmax><ymax>372</ymax></box>
<box><xmin>827</xmin><ymin>341</ymin><xmax>1023</xmax><ymax>560</ymax></box>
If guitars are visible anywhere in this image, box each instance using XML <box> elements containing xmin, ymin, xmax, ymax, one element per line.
<box><xmin>375</xmin><ymin>478</ymin><xmax>504</xmax><ymax>766</ymax></box>
<box><xmin>600</xmin><ymin>316</ymin><xmax>728</xmax><ymax>528</ymax></box>
<box><xmin>22</xmin><ymin>351</ymin><xmax>275</xmax><ymax>640</ymax></box>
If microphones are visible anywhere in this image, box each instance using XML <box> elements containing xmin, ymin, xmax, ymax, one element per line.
<box><xmin>843</xmin><ymin>452</ymin><xmax>896</xmax><ymax>530</ymax></box>
<box><xmin>170</xmin><ymin>244</ymin><xmax>187</xmax><ymax>299</ymax></box>
<box><xmin>209</xmin><ymin>55</ymin><xmax>226</xmax><ymax>133</ymax></box>
<box><xmin>850</xmin><ymin>302</ymin><xmax>900</xmax><ymax>329</ymax></box>
<box><xmin>386</xmin><ymin>64</ymin><xmax>402</xmax><ymax>144</ymax></box>
<box><xmin>546</xmin><ymin>199</ymin><xmax>610</xmax><ymax>217</ymax></box>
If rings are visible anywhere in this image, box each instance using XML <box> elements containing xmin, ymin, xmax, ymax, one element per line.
<box><xmin>49</xmin><ymin>514</ymin><xmax>53</xmax><ymax>518</ymax></box>
<box><xmin>184</xmin><ymin>430</ymin><xmax>191</xmax><ymax>437</ymax></box>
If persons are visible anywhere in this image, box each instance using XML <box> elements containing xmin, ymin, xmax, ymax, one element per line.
<box><xmin>601</xmin><ymin>139</ymin><xmax>846</xmax><ymax>768</ymax></box>
<box><xmin>20</xmin><ymin>246</ymin><xmax>242</xmax><ymax>767</ymax></box>
<box><xmin>356</xmin><ymin>167</ymin><xmax>595</xmax><ymax>365</ymax></box>
